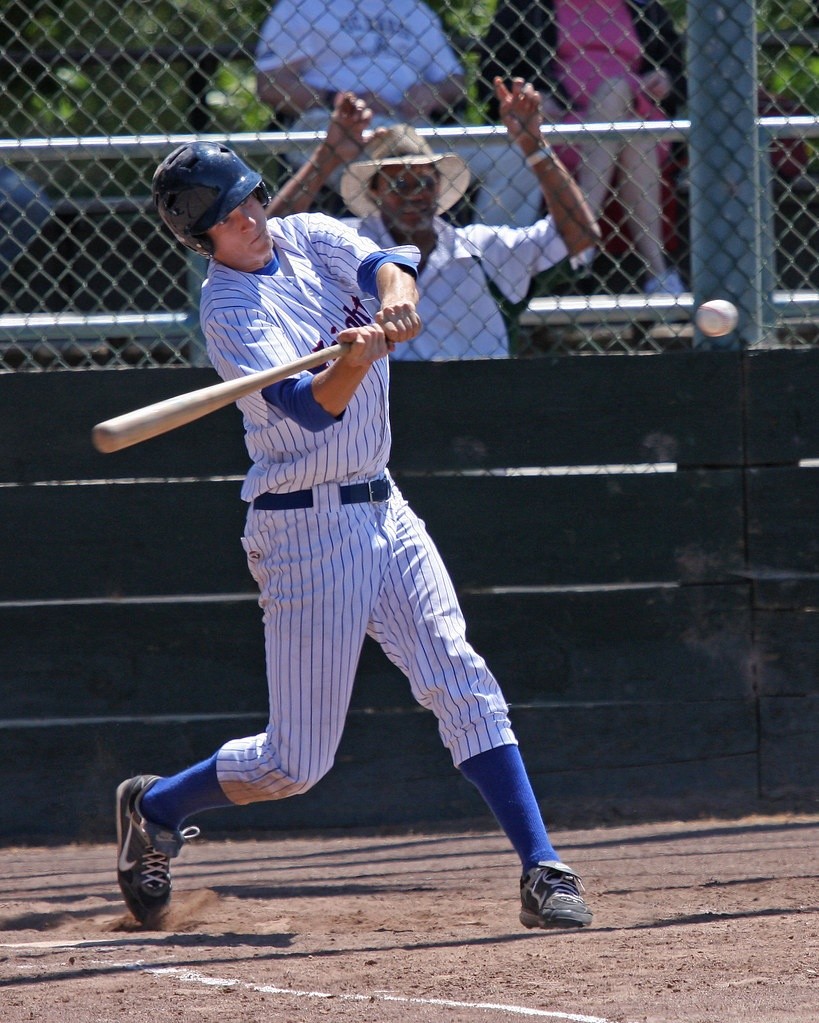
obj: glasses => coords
[384,176,437,193]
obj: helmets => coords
[150,141,271,260]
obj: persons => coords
[254,0,543,227]
[260,74,602,364]
[477,0,689,293]
[115,140,593,928]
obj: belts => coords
[254,474,392,510]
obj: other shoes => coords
[645,275,687,295]
[564,247,594,281]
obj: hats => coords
[339,124,471,218]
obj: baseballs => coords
[696,299,741,338]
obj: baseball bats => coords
[92,342,348,455]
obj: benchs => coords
[0,29,819,369]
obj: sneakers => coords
[114,775,200,924]
[519,860,592,929]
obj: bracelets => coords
[525,147,554,168]
